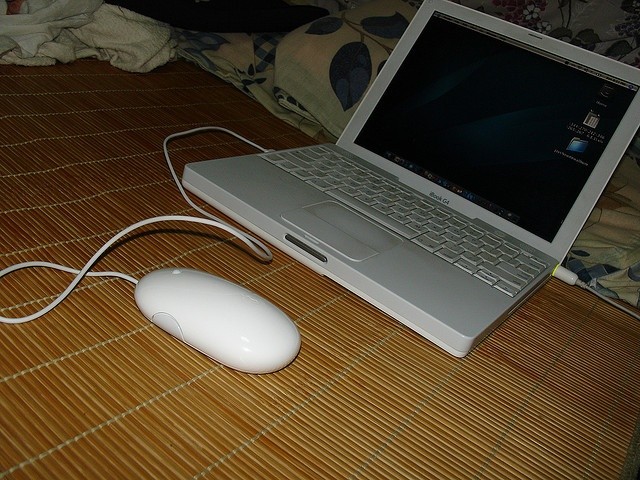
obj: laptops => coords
[180,2,640,358]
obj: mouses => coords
[136,267,302,374]
